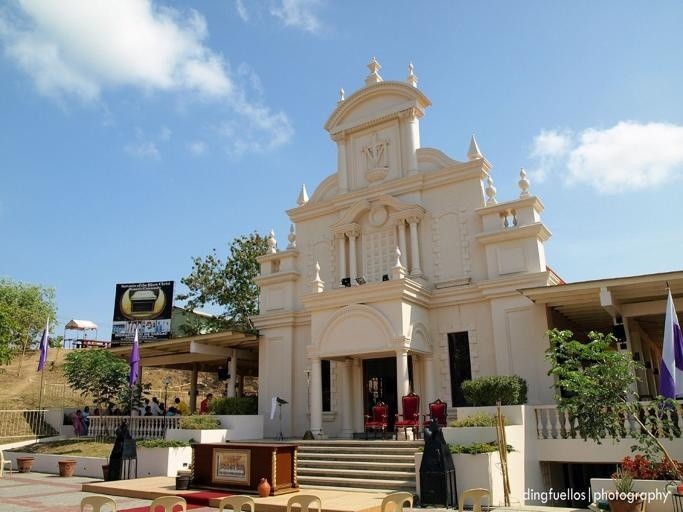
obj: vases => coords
[102,464,109,480]
[17,456,35,472]
[58,460,77,476]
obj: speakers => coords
[217,364,228,381]
[612,324,626,343]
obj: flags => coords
[37,320,49,374]
[129,326,142,389]
[659,291,683,401]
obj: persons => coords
[222,382,228,398]
[235,382,246,398]
[201,392,214,412]
[71,396,184,439]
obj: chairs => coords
[364,400,389,439]
[80,495,116,512]
[287,495,321,512]
[0,447,12,478]
[381,492,414,512]
[395,393,419,440]
[150,496,187,512]
[458,488,491,512]
[219,495,255,512]
[422,399,449,429]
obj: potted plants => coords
[607,464,646,512]
